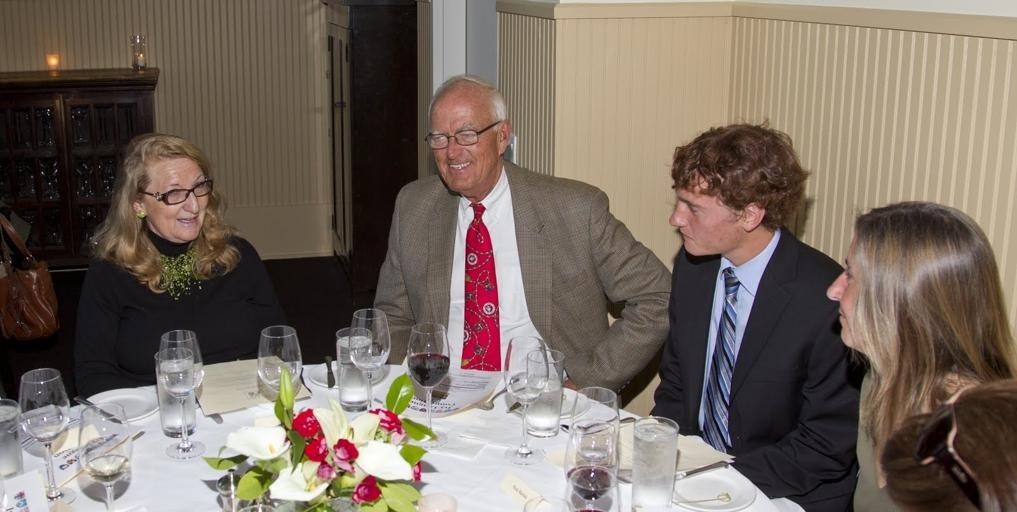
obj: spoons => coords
[559,414,635,435]
[477,375,520,411]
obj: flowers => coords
[203,366,440,512]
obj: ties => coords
[703,268,750,454]
[461,203,501,371]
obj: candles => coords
[48,56,58,71]
[137,53,146,68]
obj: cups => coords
[525,349,566,439]
[153,347,198,437]
[418,491,460,511]
[524,496,570,510]
[335,327,373,411]
[631,416,679,511]
[0,397,25,482]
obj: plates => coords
[308,361,383,389]
[671,472,755,511]
[86,388,159,424]
[558,388,589,420]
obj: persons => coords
[826,202,1017,512]
[649,120,870,512]
[881,377,1017,512]
[373,73,672,400]
[75,132,289,403]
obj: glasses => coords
[139,179,213,205]
[915,383,999,512]
[424,120,500,150]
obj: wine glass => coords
[502,335,546,466]
[18,368,77,508]
[349,308,392,425]
[256,325,302,424]
[78,401,130,511]
[159,329,206,460]
[0,108,117,246]
[563,384,623,512]
[406,322,450,451]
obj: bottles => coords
[217,468,243,512]
[128,33,147,75]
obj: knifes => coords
[324,353,335,389]
[74,395,123,426]
[675,458,730,479]
[503,400,520,414]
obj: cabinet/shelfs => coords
[1,68,160,269]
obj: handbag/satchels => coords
[0,212,61,340]
